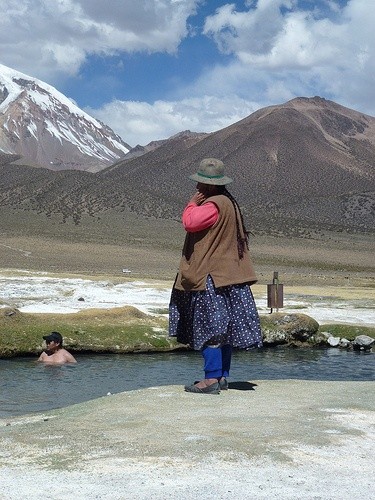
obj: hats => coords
[189,157,233,185]
[43,331,63,343]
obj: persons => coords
[169,158,263,394]
[38,332,77,363]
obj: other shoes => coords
[194,378,228,390]
[184,381,220,394]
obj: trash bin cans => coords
[267,271,283,313]
